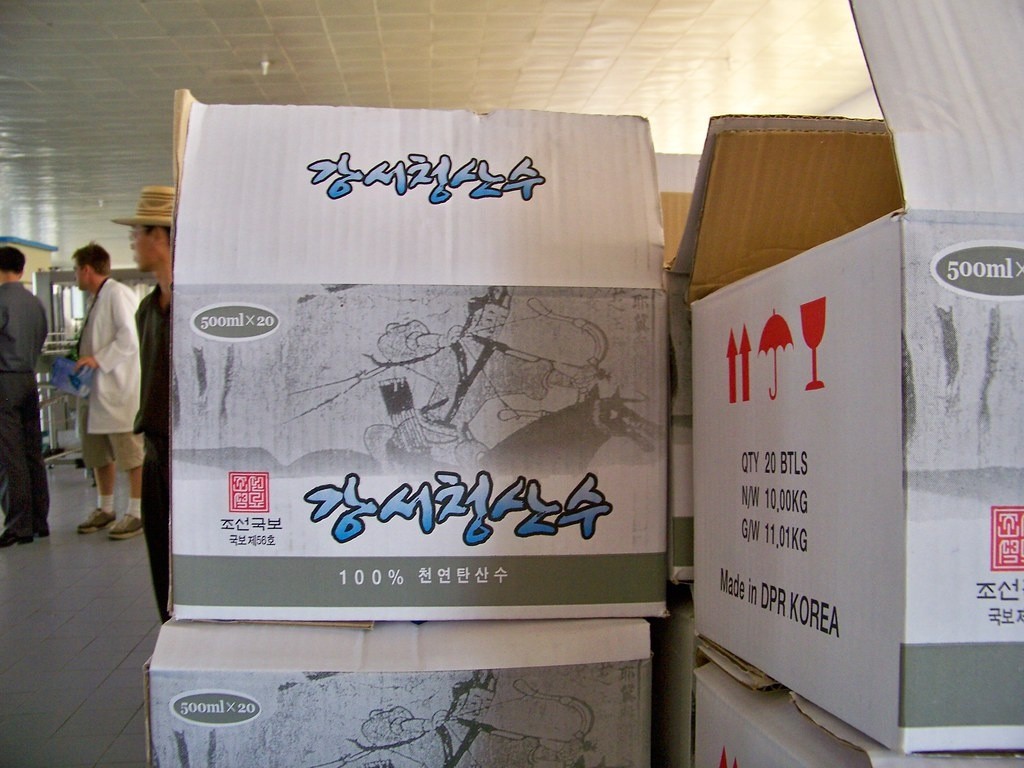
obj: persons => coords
[69,244,147,540]
[110,184,176,622]
[0,247,51,549]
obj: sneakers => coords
[108,514,144,539]
[77,508,115,534]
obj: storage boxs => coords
[142,618,654,768]
[656,115,890,587]
[680,0,1024,759]
[691,645,1024,768]
[165,89,673,629]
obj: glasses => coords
[128,228,157,241]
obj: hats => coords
[110,185,179,226]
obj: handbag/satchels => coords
[48,357,94,399]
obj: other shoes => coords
[33,518,49,537]
[0,529,34,548]
